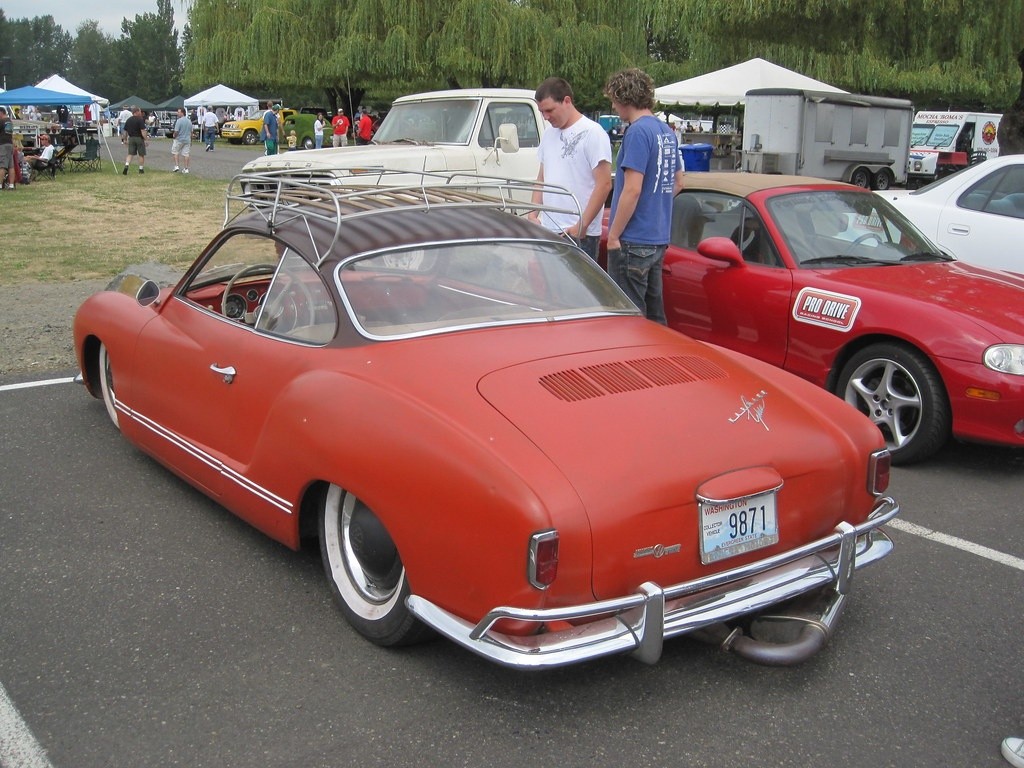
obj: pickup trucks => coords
[221,110,297,146]
[299,106,332,122]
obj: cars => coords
[596,172,1024,468]
[72,161,897,670]
[157,113,198,138]
[278,114,348,150]
[831,153,1024,276]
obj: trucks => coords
[238,87,617,225]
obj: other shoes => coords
[139,169,144,173]
[0,188,2,190]
[206,145,210,151]
[9,188,14,191]
[123,165,128,175]
[211,149,214,151]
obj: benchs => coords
[967,193,1024,219]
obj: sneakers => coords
[173,166,179,172]
[182,168,189,173]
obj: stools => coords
[712,144,731,171]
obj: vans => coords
[908,110,1004,182]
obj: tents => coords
[35,74,259,143]
[0,85,119,174]
[649,57,849,133]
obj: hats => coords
[272,104,283,110]
[38,135,51,140]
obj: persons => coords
[20,134,55,171]
[356,110,372,146]
[526,77,613,264]
[172,107,193,174]
[113,101,297,155]
[601,68,683,327]
[314,112,326,149]
[610,118,705,148]
[332,108,350,147]
[14,108,21,119]
[122,106,148,175]
[0,107,17,189]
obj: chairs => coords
[66,139,102,174]
[670,194,706,249]
[2,162,24,189]
[28,142,77,182]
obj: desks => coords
[681,133,742,172]
[61,127,98,172]
[22,147,45,157]
